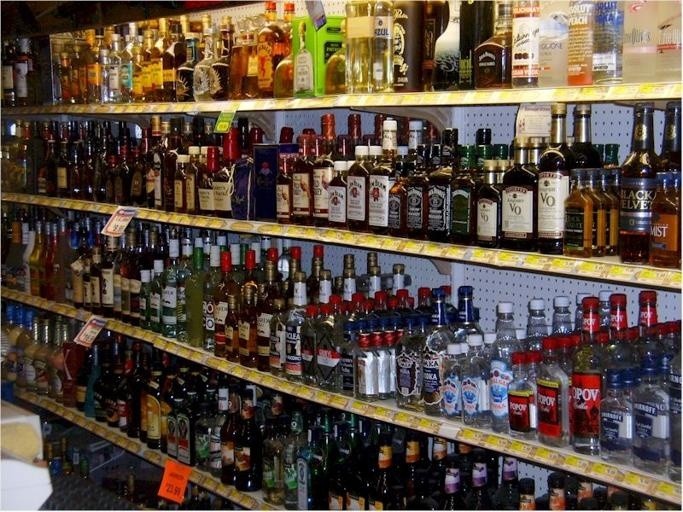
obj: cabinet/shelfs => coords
[1,0,682,511]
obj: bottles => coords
[0,1,681,511]
